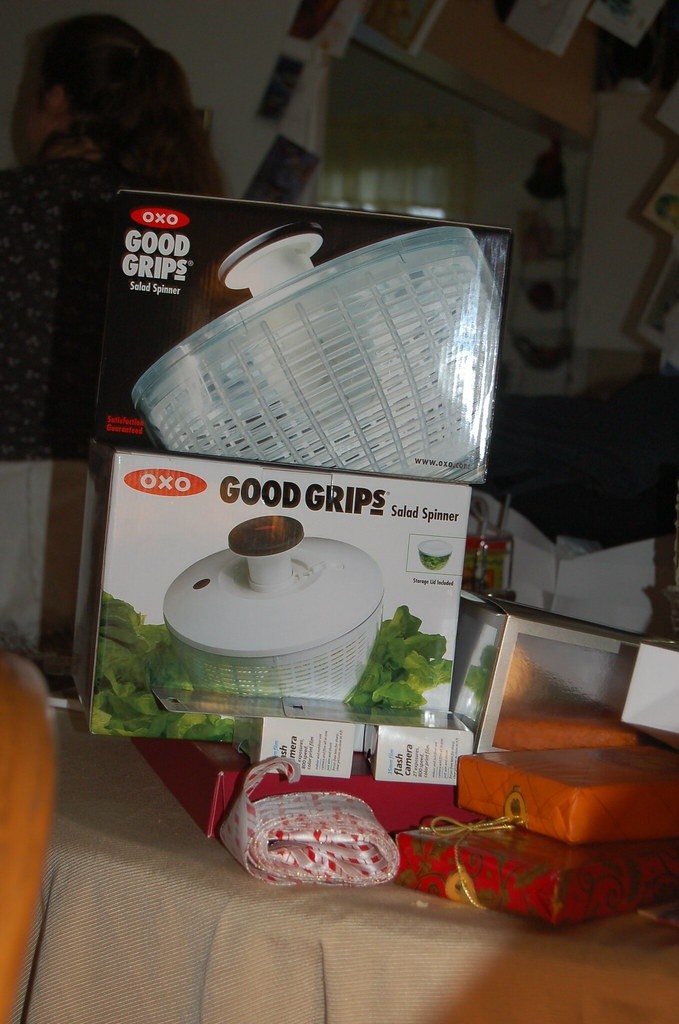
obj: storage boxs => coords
[64,188,679,927]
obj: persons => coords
[0,12,225,462]
